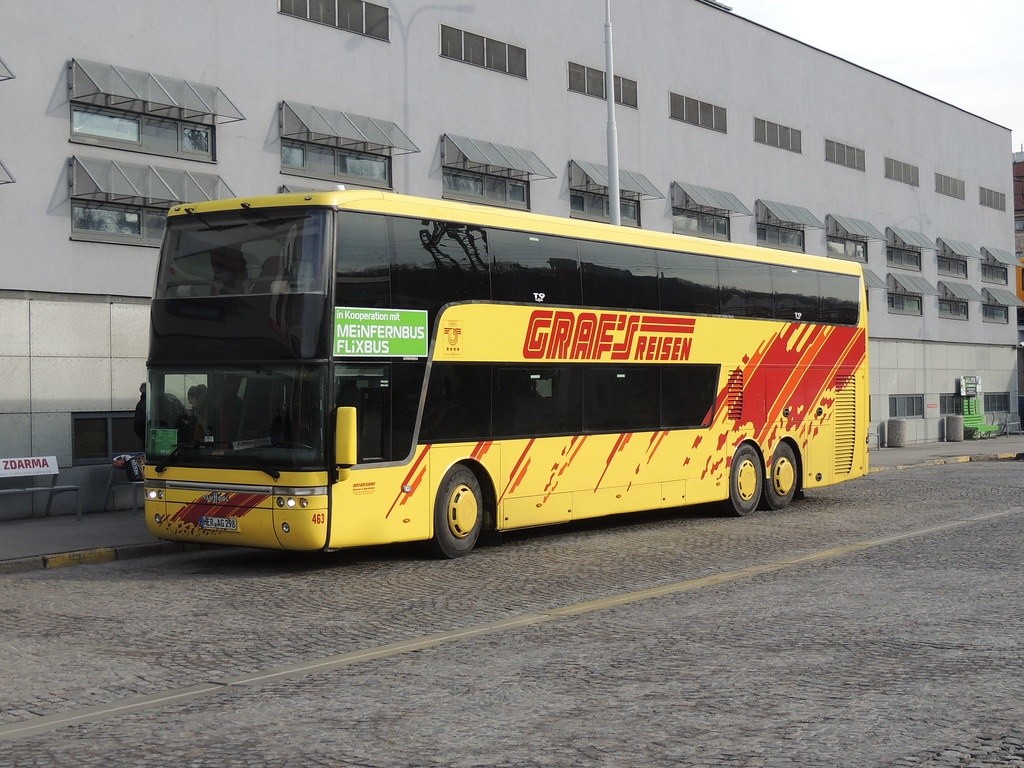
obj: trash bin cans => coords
[887,418,907,448]
[946,415,963,442]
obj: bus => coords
[142,187,870,563]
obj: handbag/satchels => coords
[124,453,145,482]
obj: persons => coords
[134,381,227,452]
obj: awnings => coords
[569,159,666,201]
[983,287,1024,306]
[280,100,421,156]
[441,133,557,180]
[70,155,239,211]
[939,281,988,302]
[981,246,1024,268]
[936,237,987,260]
[862,268,890,290]
[888,272,942,297]
[671,180,754,217]
[825,213,888,242]
[756,199,827,230]
[885,225,941,251]
[71,57,247,125]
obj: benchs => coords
[987,411,1022,437]
[962,415,999,441]
[101,453,144,517]
[0,456,84,522]
